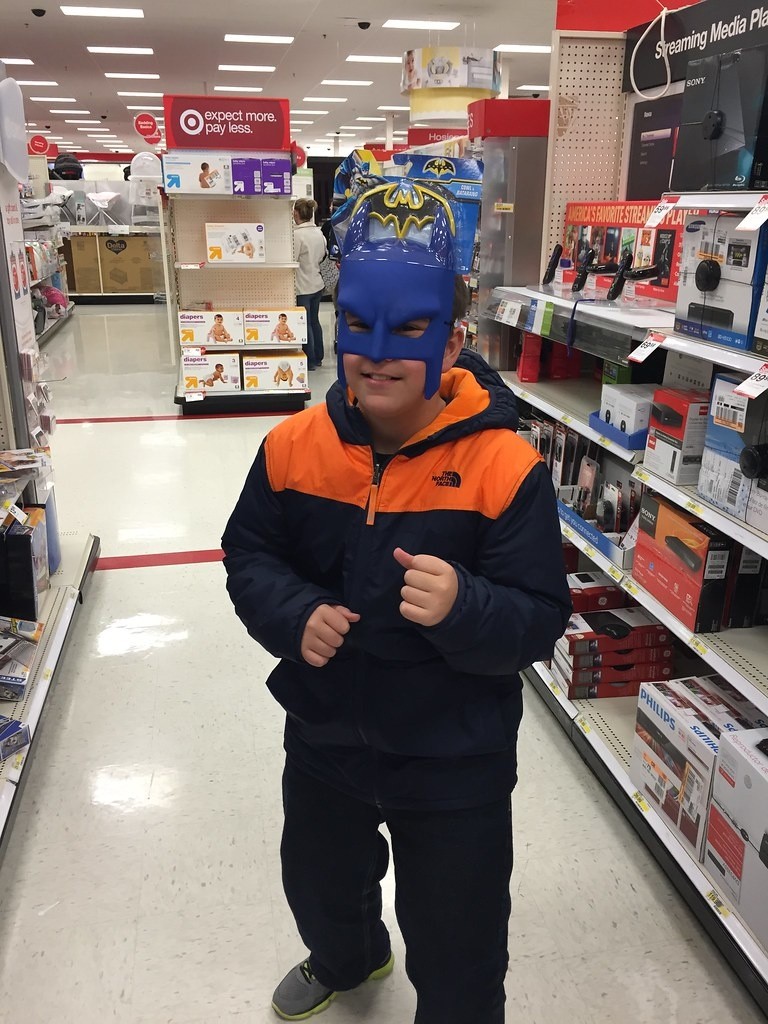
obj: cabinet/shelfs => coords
[167,193,313,415]
[22,181,79,353]
[487,288,768,1015]
[1,159,101,837]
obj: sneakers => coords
[270,946,394,1020]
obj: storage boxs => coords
[0,503,50,763]
[515,191,767,959]
[163,154,309,394]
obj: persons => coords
[199,363,228,387]
[221,181,569,1023]
[207,314,233,343]
[271,314,296,342]
[274,361,294,387]
[403,50,421,90]
[237,242,254,259]
[199,163,217,188]
[292,198,328,371]
